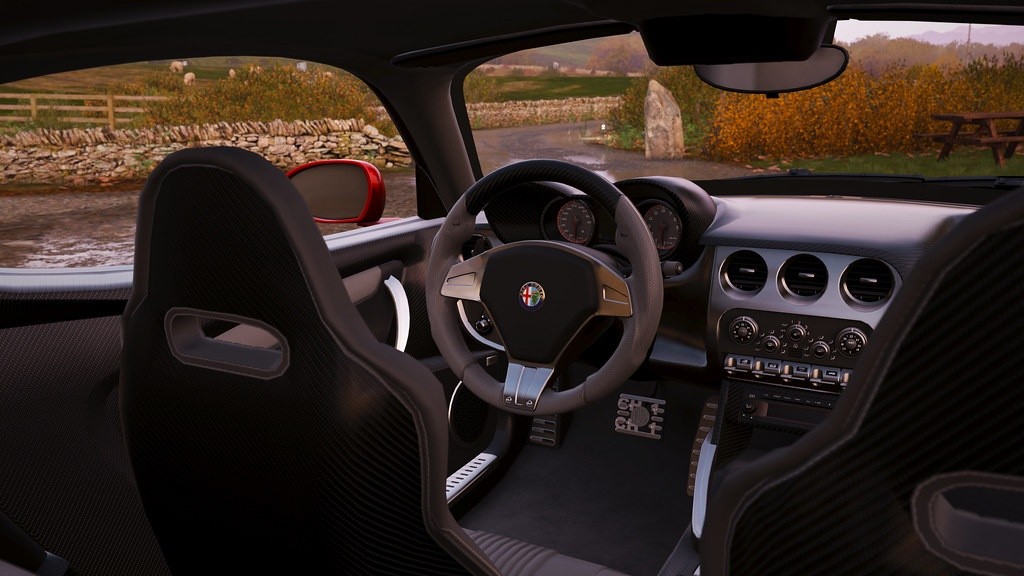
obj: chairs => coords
[697,196,1024,576]
[118,146,625,576]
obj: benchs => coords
[965,136,1024,143]
[916,129,1016,138]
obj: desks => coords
[930,112,1024,166]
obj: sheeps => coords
[229,69,236,78]
[169,62,183,74]
[184,72,196,86]
